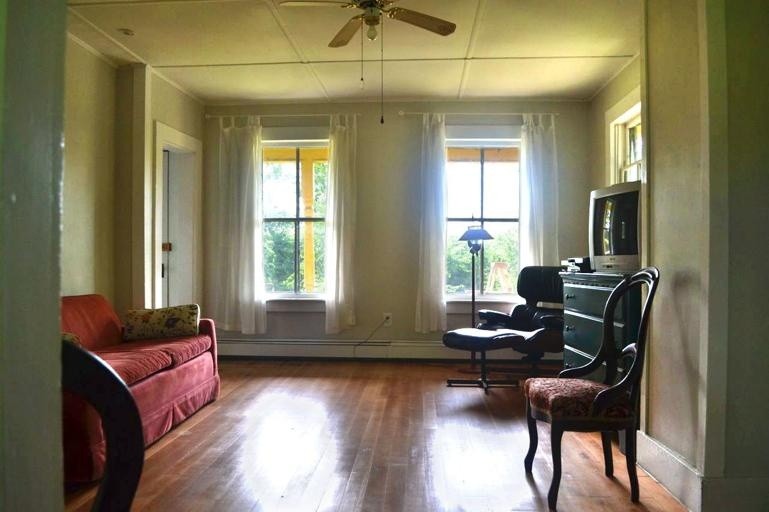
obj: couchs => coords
[59,294,222,493]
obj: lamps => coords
[356,2,389,125]
[456,222,493,373]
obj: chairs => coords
[520,265,659,512]
[440,263,566,394]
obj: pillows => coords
[120,303,201,341]
[60,331,80,343]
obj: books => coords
[537,300,564,310]
[560,255,590,265]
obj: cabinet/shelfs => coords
[558,266,643,456]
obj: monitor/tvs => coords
[589,180,641,274]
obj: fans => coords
[278,1,458,50]
